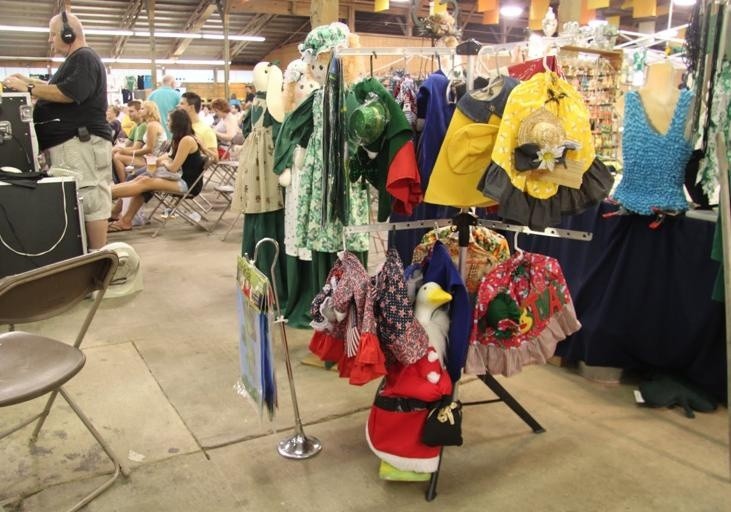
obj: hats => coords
[349,101,385,146]
[298,23,350,60]
[517,106,568,175]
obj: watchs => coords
[25,83,35,96]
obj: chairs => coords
[0,250,130,512]
[148,141,243,241]
[375,0,658,37]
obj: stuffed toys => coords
[409,281,453,370]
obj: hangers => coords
[344,219,530,285]
[356,48,573,93]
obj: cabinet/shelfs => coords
[559,41,619,167]
[345,214,596,502]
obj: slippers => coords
[108,221,133,233]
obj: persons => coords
[227,60,286,214]
[0,7,116,253]
[270,54,322,263]
[103,73,257,235]
[607,59,699,218]
[268,22,367,256]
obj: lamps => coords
[0,25,265,42]
[52,57,231,65]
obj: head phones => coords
[61,11,76,44]
